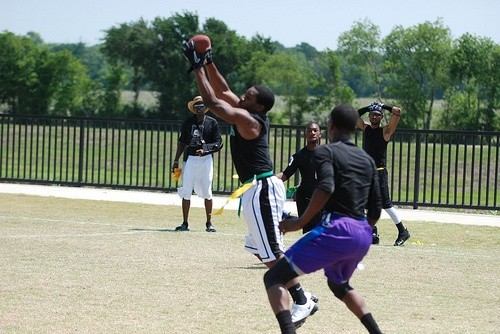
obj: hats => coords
[369,105,383,114]
[188,96,209,114]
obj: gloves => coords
[180,40,211,74]
[367,102,384,112]
[205,49,212,65]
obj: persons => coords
[263,103,382,334]
[180,39,319,332]
[170,95,223,232]
[355,102,410,247]
[275,120,330,234]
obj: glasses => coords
[193,104,204,108]
[370,113,381,116]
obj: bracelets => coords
[173,160,179,164]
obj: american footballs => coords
[187,34,210,57]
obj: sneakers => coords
[206,222,216,232]
[372,226,379,244]
[282,210,291,221]
[394,228,410,246]
[175,222,188,231]
[290,291,318,329]
